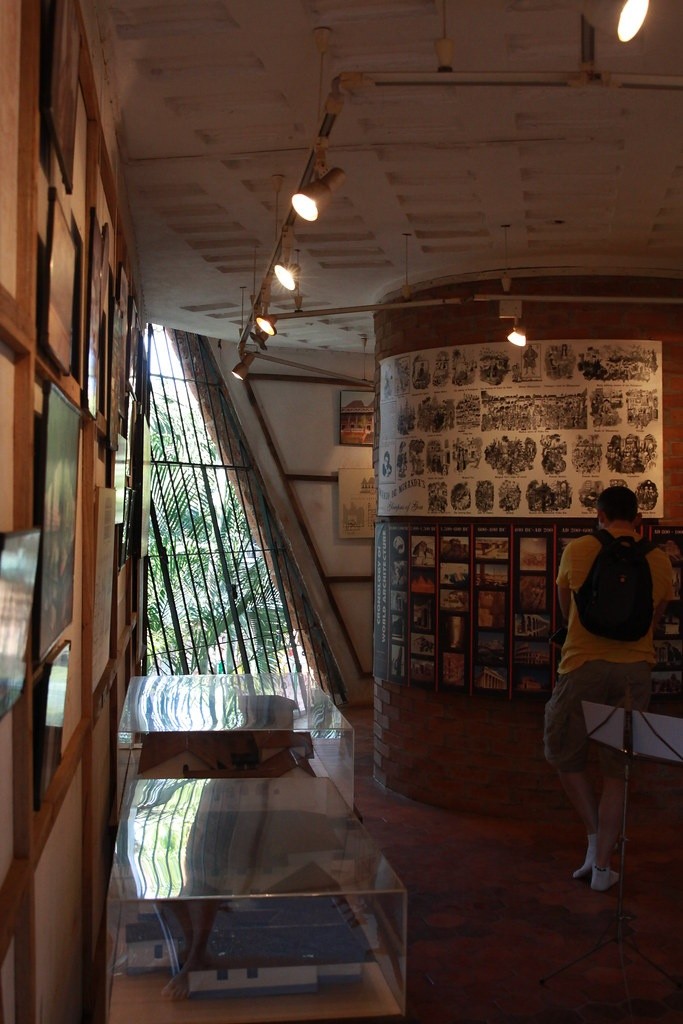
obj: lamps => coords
[506,320,530,348]
[256,313,278,337]
[289,163,349,224]
[273,261,302,292]
[582,0,651,45]
[231,354,256,381]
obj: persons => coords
[543,484,673,892]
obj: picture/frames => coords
[33,382,84,669]
[38,0,140,449]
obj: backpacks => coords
[573,528,657,641]
